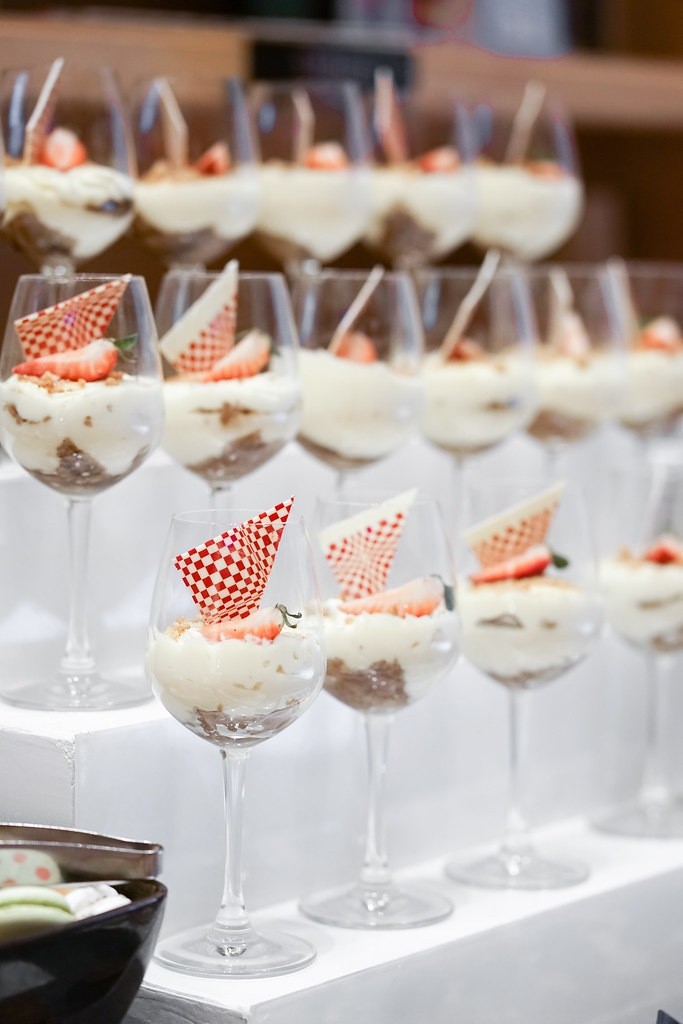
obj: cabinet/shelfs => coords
[0,440,683,1024]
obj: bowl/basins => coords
[0,879,170,1024]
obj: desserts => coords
[0,127,682,753]
[0,881,131,948]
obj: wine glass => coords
[2,263,683,721]
[0,52,588,342]
[151,484,683,977]
